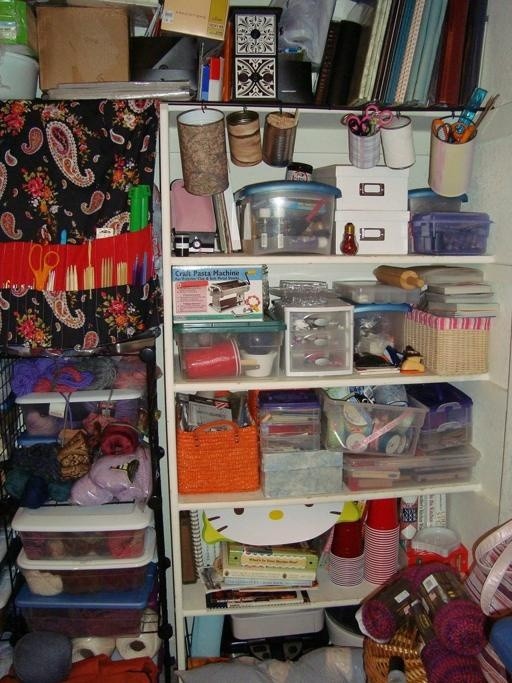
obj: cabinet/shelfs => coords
[0,330,173,682]
[171,255,494,617]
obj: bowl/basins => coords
[322,610,367,650]
[2,47,42,102]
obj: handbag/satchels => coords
[460,520,512,681]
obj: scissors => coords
[28,244,60,291]
[432,117,474,144]
[342,105,393,136]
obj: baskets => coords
[362,621,428,682]
[399,309,489,375]
[177,414,259,495]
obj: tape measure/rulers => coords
[349,407,414,448]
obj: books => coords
[179,512,222,583]
[158,0,490,109]
[415,267,500,320]
[221,546,316,588]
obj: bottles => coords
[175,234,190,256]
[339,222,357,254]
[285,161,314,183]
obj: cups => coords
[347,115,379,170]
[380,114,415,170]
[425,116,477,199]
[261,110,298,166]
[329,497,401,587]
[176,107,230,195]
[181,332,279,378]
[226,110,263,167]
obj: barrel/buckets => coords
[325,609,364,647]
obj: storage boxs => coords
[11,501,158,638]
[16,351,146,438]
[232,164,494,254]
[255,382,481,499]
[36,6,131,92]
[173,265,411,378]
